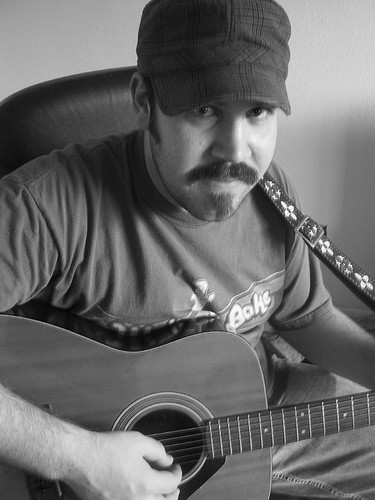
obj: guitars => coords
[0,312,375,496]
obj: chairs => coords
[0,66,145,177]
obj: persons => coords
[0,1,375,500]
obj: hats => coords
[136,1,292,116]
